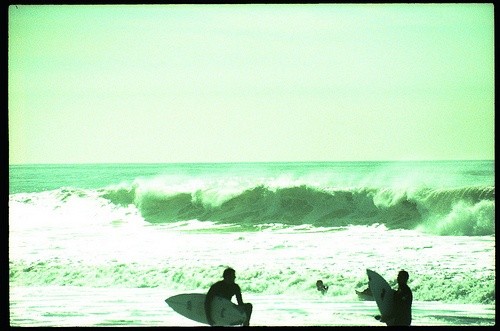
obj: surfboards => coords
[165,294,246,327]
[355,290,374,301]
[367,268,392,317]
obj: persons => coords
[205,268,253,327]
[375,271,413,326]
[316,280,329,295]
